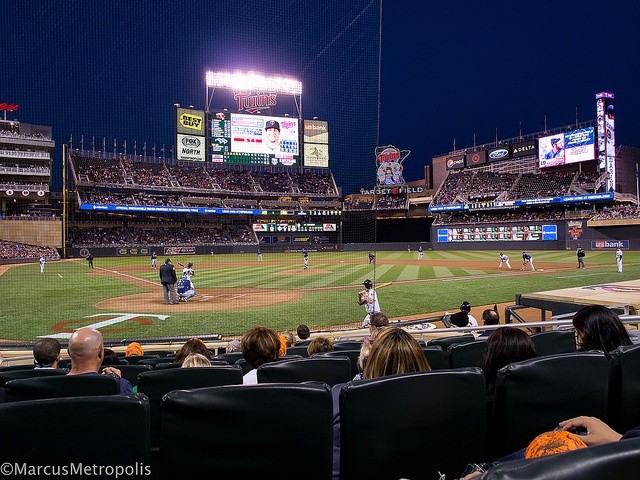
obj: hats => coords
[551,137,564,146]
[265,119,281,133]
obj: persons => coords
[151,252,157,266]
[572,305,634,353]
[177,262,195,303]
[87,253,93,267]
[369,252,376,264]
[159,257,177,304]
[40,256,45,272]
[481,308,501,337]
[303,250,308,269]
[521,253,535,271]
[298,324,311,341]
[616,247,623,273]
[417,245,423,260]
[576,244,587,269]
[306,337,335,356]
[330,327,431,480]
[257,249,262,260]
[498,253,513,269]
[34,338,61,369]
[282,331,295,349]
[358,279,381,328]
[177,338,213,366]
[450,301,479,330]
[545,139,565,159]
[67,328,132,392]
[241,326,282,385]
[452,416,639,480]
[363,314,390,337]
[263,121,282,154]
[482,328,540,397]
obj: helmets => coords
[460,300,472,312]
[363,279,374,289]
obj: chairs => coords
[333,342,362,351]
[137,365,243,403]
[138,358,175,364]
[427,334,475,348]
[421,346,445,370]
[159,381,334,480]
[488,352,609,460]
[449,340,488,369]
[0,392,152,479]
[530,330,576,357]
[235,356,294,375]
[485,436,640,479]
[310,350,360,378]
[609,342,640,435]
[286,347,309,360]
[339,367,489,479]
[118,355,160,364]
[3,374,120,405]
[210,354,244,365]
[257,355,352,386]
[0,369,70,386]
[102,364,153,382]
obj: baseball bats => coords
[358,282,392,294]
[177,263,186,268]
